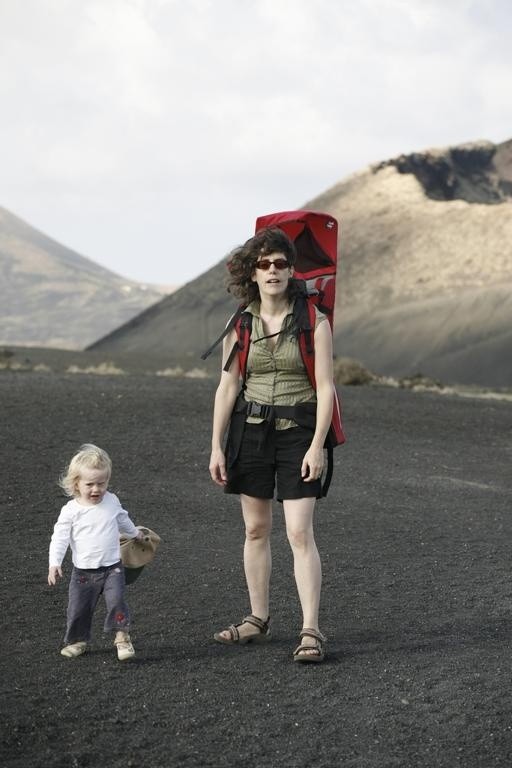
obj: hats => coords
[120,526,160,584]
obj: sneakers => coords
[60,641,88,657]
[115,633,134,660]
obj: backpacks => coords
[237,209,348,451]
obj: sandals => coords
[214,614,275,644]
[295,627,325,665]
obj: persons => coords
[45,442,147,662]
[205,223,338,665]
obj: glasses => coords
[255,260,292,271]
